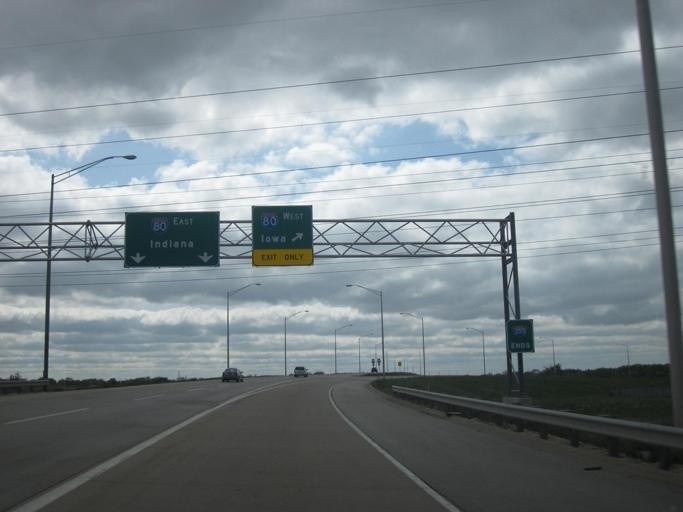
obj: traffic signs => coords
[126,212,220,267]
[252,205,315,267]
[506,319,535,353]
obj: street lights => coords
[335,321,354,374]
[351,330,429,373]
[616,342,631,366]
[44,153,138,380]
[466,327,486,375]
[344,281,387,380]
[284,309,309,376]
[226,281,263,369]
[541,336,556,367]
[398,309,428,376]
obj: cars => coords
[221,367,244,383]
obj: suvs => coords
[293,365,309,378]
